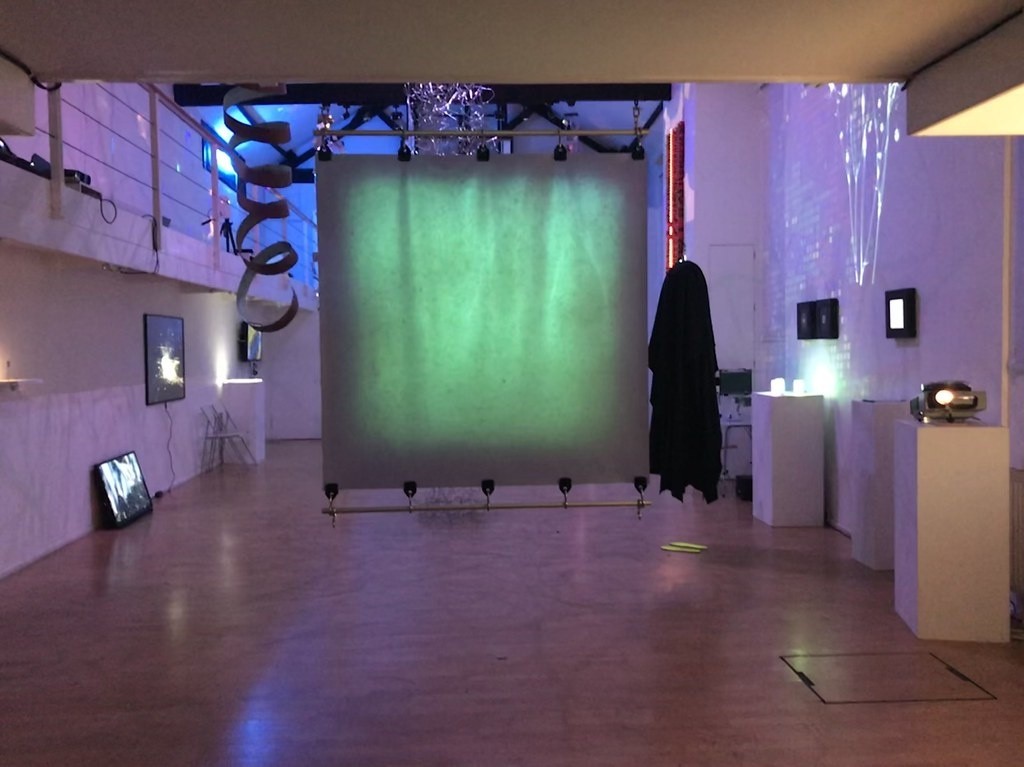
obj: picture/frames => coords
[885,289,916,339]
[662,120,685,270]
[795,298,839,342]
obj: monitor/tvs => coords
[240,322,261,362]
[95,451,153,529]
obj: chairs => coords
[198,401,257,481]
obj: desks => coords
[720,420,755,489]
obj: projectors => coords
[909,389,986,423]
